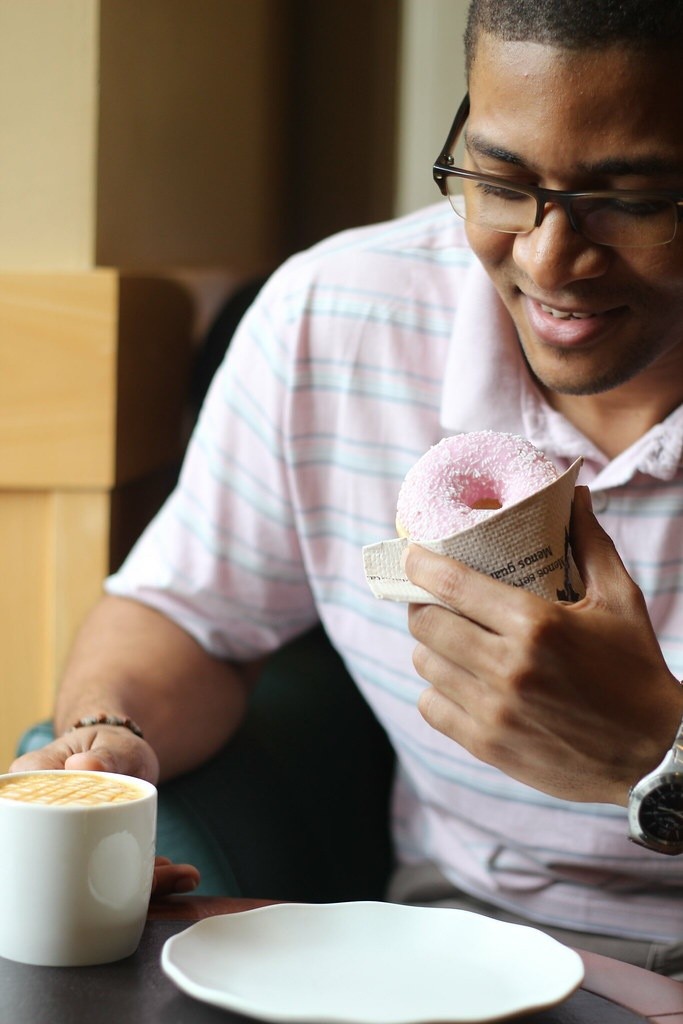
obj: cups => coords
[1,771,159,970]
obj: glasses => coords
[432,91,683,248]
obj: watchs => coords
[628,711,683,856]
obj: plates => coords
[160,901,586,1024]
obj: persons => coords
[8,0,683,982]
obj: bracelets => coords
[62,713,144,740]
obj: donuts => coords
[394,430,559,543]
